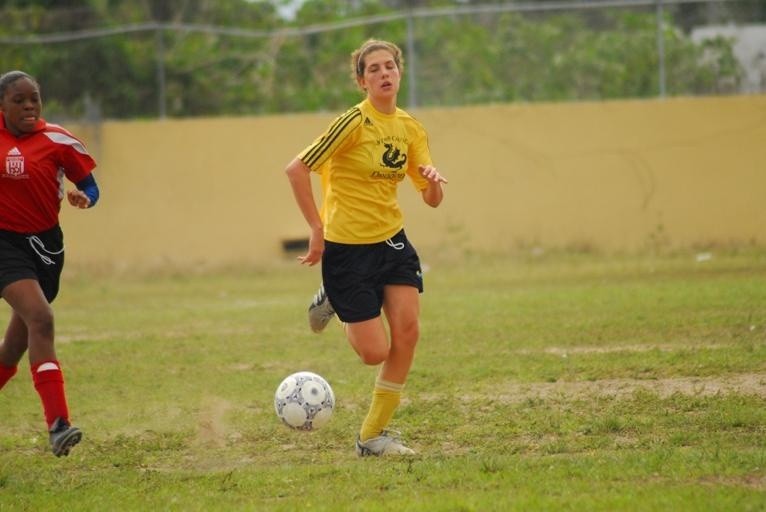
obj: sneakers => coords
[355,428,416,456]
[49,417,83,458]
[308,282,335,335]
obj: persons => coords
[286,38,449,458]
[0,70,103,459]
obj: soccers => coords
[274,371,334,432]
